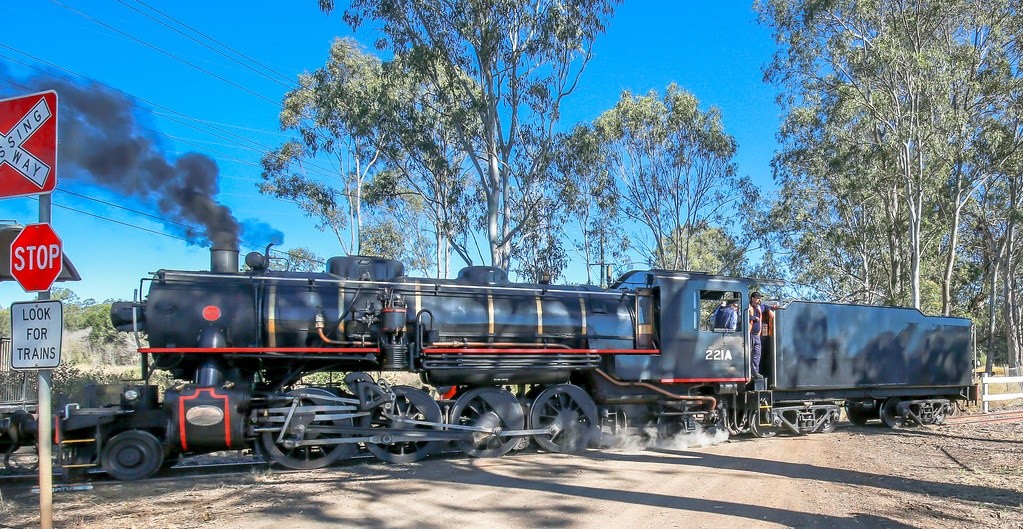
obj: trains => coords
[0,238,987,483]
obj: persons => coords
[710,298,739,331]
[749,292,782,379]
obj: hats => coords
[727,299,739,304]
[751,292,764,297]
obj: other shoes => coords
[753,373,764,379]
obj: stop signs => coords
[9,222,65,293]
